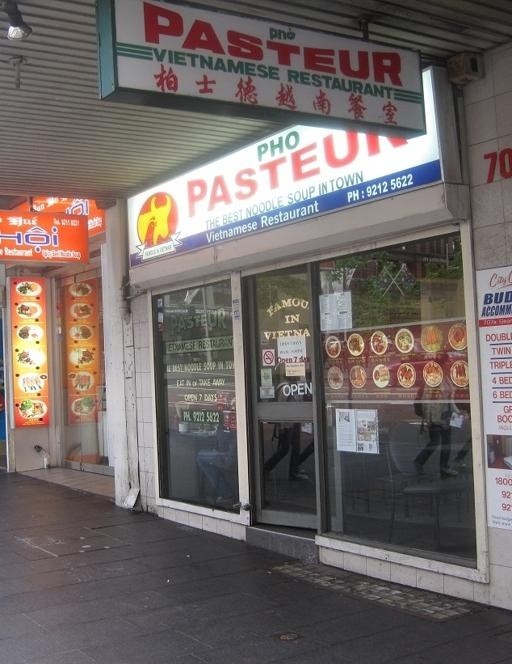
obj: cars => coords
[382,352,466,388]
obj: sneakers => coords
[413,458,427,475]
[215,495,235,503]
[453,454,466,467]
[288,471,309,480]
[440,469,458,476]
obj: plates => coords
[325,323,470,390]
[16,281,48,420]
[69,282,98,417]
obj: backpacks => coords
[414,381,428,435]
[267,384,285,424]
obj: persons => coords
[196,433,238,504]
[413,352,457,477]
[455,438,473,467]
[265,364,308,481]
